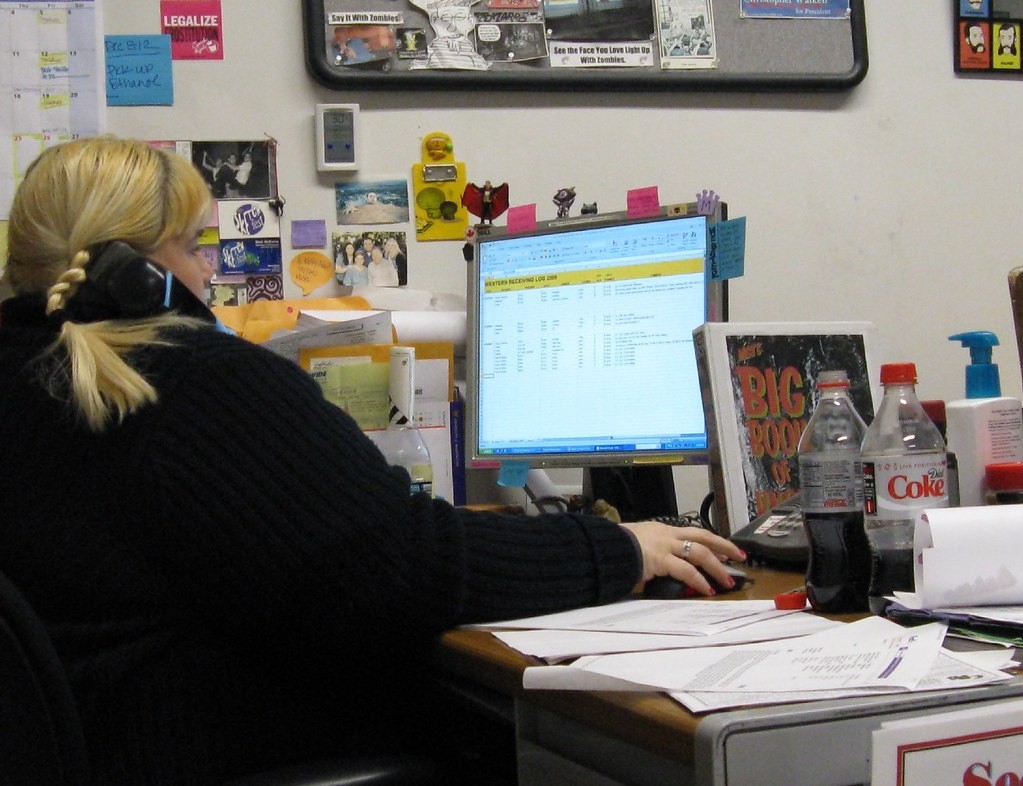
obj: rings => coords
[682,539,691,558]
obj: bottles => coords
[798,371,869,616]
[372,420,433,498]
[984,462,1023,504]
[899,400,960,507]
[859,363,949,617]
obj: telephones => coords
[74,237,219,325]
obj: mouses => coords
[642,560,746,601]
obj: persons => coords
[471,181,504,224]
[331,27,375,65]
[202,147,253,197]
[336,232,407,286]
[0,137,746,786]
[367,191,377,202]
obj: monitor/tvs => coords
[463,201,729,527]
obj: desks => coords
[410,560,1022,786]
